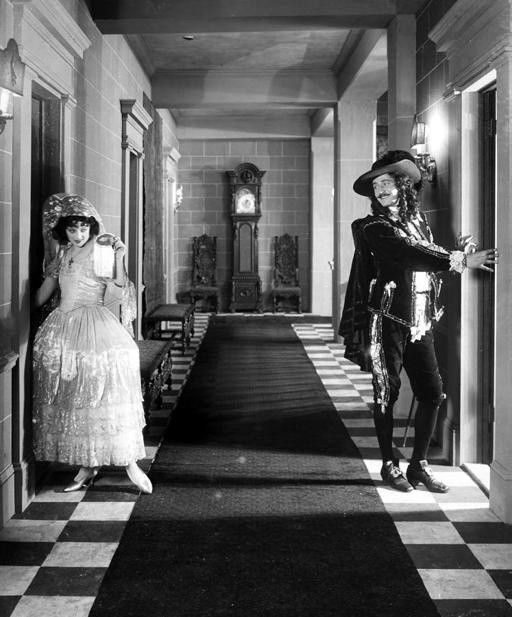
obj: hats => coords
[353,158,421,197]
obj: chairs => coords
[188,232,220,314]
[267,233,307,315]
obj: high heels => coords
[406,460,449,494]
[126,465,152,493]
[381,464,414,492]
[64,467,97,491]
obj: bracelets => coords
[464,255,468,268]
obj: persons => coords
[33,193,153,494]
[337,152,499,493]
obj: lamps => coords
[410,113,437,184]
[0,37,26,133]
[173,183,184,214]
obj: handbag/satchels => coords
[93,232,115,278]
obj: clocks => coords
[224,162,269,315]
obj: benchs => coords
[150,302,196,352]
[133,338,177,417]
